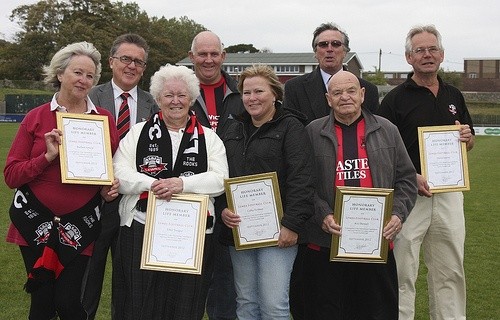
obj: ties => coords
[116,92,131,141]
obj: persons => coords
[213,63,317,320]
[302,69,419,320]
[283,21,381,127]
[3,41,122,320]
[79,30,159,320]
[110,62,231,320]
[376,22,477,320]
[186,29,240,134]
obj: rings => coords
[164,187,168,191]
[396,227,400,233]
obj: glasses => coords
[411,46,441,55]
[112,55,147,67]
[314,39,348,49]
